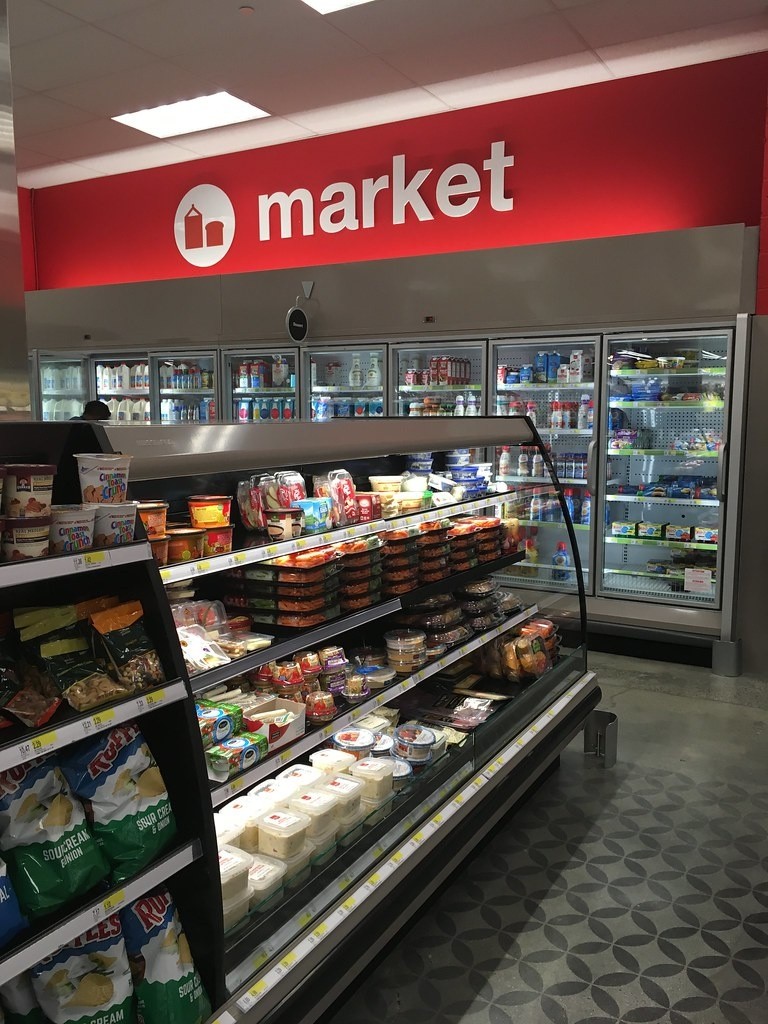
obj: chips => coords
[44,952,118,1009]
[161,928,193,964]
[14,793,73,829]
[113,765,166,798]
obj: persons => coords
[68,401,111,420]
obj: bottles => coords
[365,353,382,386]
[160,363,214,390]
[160,397,216,421]
[42,397,88,421]
[498,442,589,480]
[96,363,150,389]
[506,527,571,580]
[348,353,363,387]
[496,394,594,430]
[503,483,591,526]
[41,366,81,391]
[605,502,611,526]
[607,456,613,480]
[408,395,482,418]
[98,396,150,423]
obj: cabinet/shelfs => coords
[30,312,752,678]
[0,415,620,1024]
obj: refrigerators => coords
[30,313,751,640]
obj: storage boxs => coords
[354,494,381,522]
[646,548,717,581]
[611,520,718,543]
[242,698,306,753]
[289,496,333,535]
